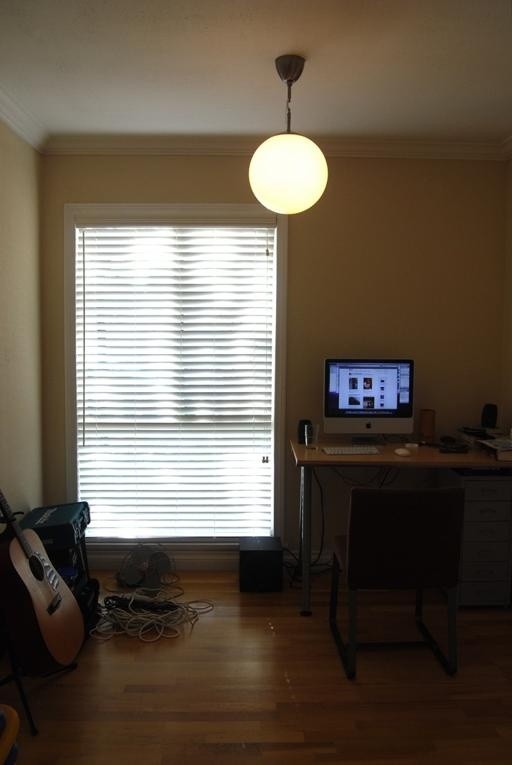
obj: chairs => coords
[325,486,464,680]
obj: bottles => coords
[420,409,436,443]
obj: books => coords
[476,438,512,461]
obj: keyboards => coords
[322,445,381,455]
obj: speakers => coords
[298,420,313,444]
[481,403,498,428]
[239,537,283,592]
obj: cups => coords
[305,424,319,449]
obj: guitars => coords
[2,493,87,673]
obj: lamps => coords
[248,53,330,216]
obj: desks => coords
[289,437,511,617]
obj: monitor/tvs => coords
[322,354,415,444]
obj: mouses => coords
[394,449,411,456]
